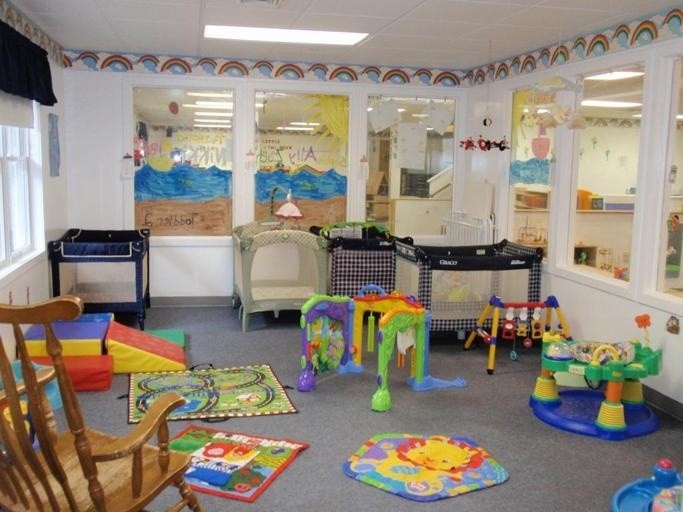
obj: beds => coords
[311,222,413,300]
[392,239,544,340]
[398,210,492,299]
[48,228,150,331]
[232,219,328,333]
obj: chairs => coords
[0,295,205,511]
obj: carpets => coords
[168,426,310,502]
[344,433,510,501]
[127,364,298,424]
